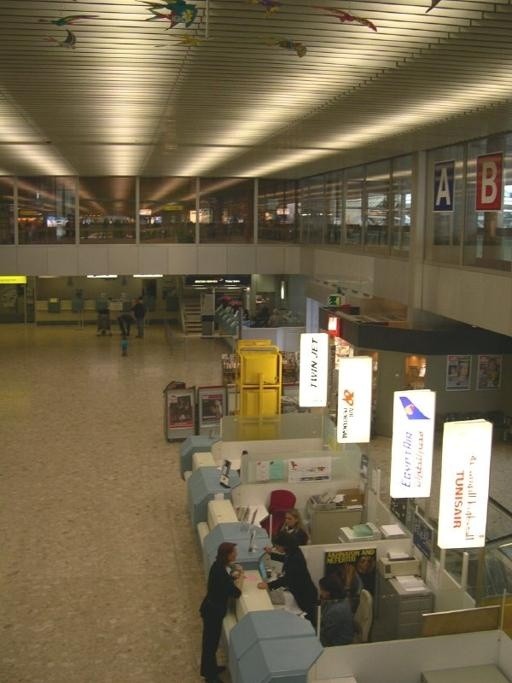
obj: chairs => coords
[317,563,375,643]
[260,490,298,538]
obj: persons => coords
[278,510,308,545]
[199,543,244,683]
[9,214,193,244]
[319,577,354,646]
[242,295,286,326]
[484,359,499,384]
[95,297,147,355]
[330,555,372,598]
[208,399,223,415]
[169,401,192,425]
[449,361,469,386]
[258,532,317,623]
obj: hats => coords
[219,542,236,553]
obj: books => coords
[396,575,425,591]
[379,525,404,538]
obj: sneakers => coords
[200,664,225,683]
